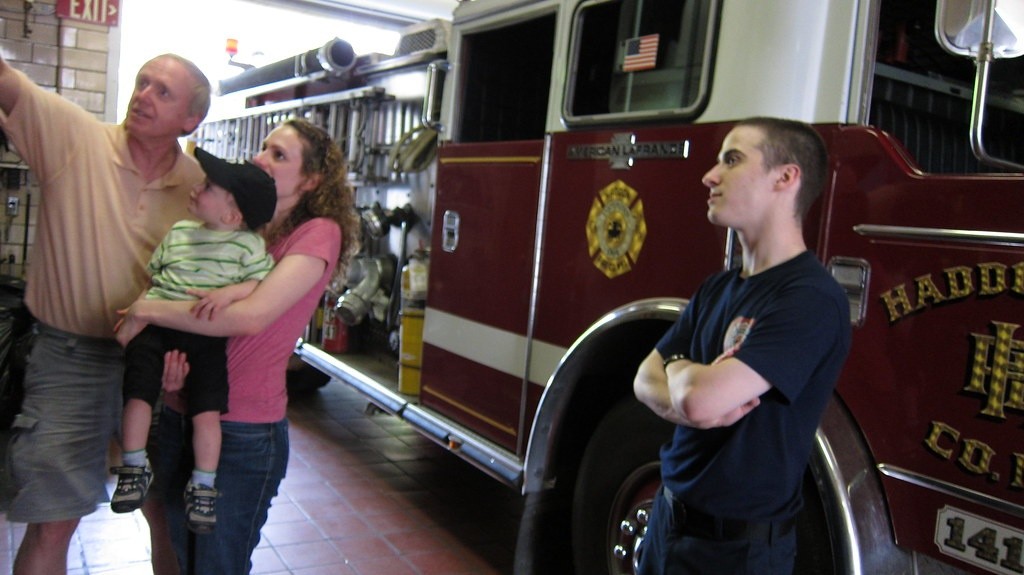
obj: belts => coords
[663,486,797,541]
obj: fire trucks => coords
[182,0,1024,574]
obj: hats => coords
[195,147,277,230]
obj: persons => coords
[634,117,851,575]
[113,120,357,575]
[110,146,277,527]
[0,56,210,575]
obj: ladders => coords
[197,86,386,188]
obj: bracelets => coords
[663,353,687,372]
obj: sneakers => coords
[183,479,218,533]
[110,459,154,514]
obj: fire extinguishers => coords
[323,292,348,354]
